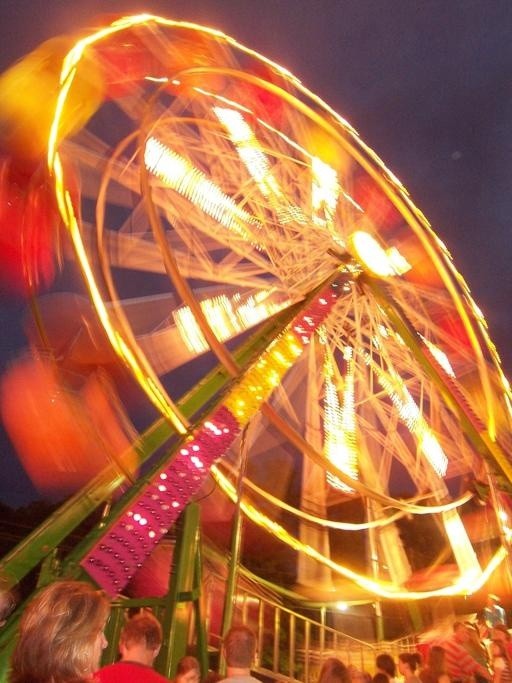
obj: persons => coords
[0,573,263,683]
[317,593,512,683]
